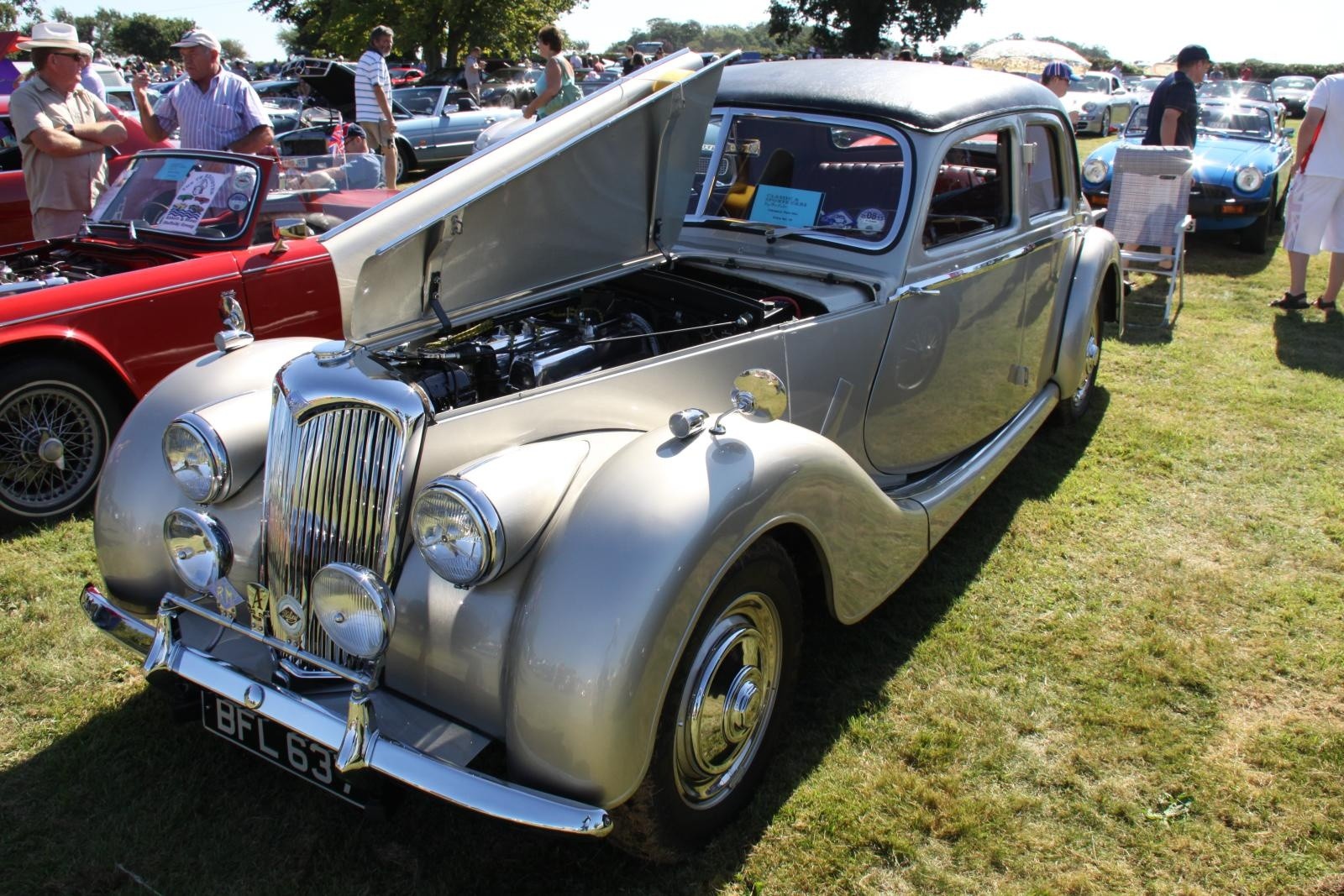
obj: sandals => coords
[1268,293,1310,309]
[1313,296,1336,313]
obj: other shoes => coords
[1154,266,1172,273]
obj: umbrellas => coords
[972,42,1092,77]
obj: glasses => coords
[47,51,81,62]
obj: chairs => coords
[1110,145,1191,333]
[1229,115,1262,129]
[408,96,432,113]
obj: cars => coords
[1196,80,1287,129]
[692,118,998,192]
[1122,75,1165,106]
[1082,98,1294,256]
[1270,75,1317,120]
[0,149,402,532]
[1059,70,1141,138]
[0,57,623,248]
[79,47,1127,860]
[635,42,663,65]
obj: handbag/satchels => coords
[544,58,582,114]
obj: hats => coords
[342,122,367,139]
[15,22,92,56]
[1043,63,1083,82]
[1178,45,1214,64]
[170,29,221,53]
[78,43,94,58]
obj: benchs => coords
[812,157,1007,240]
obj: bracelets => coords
[224,147,232,152]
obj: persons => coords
[220,58,280,80]
[355,25,397,190]
[297,80,316,106]
[465,46,486,107]
[1112,61,1252,80]
[327,123,344,165]
[617,46,665,77]
[7,22,129,239]
[132,29,275,224]
[154,59,183,82]
[1121,44,1210,275]
[419,53,447,73]
[115,62,124,79]
[708,46,971,66]
[339,55,344,62]
[523,26,574,122]
[123,56,157,77]
[524,51,606,80]
[1268,73,1344,309]
[288,50,295,61]
[94,48,113,67]
[298,123,380,189]
[1025,62,1084,216]
[78,42,106,103]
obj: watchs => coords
[64,124,75,136]
